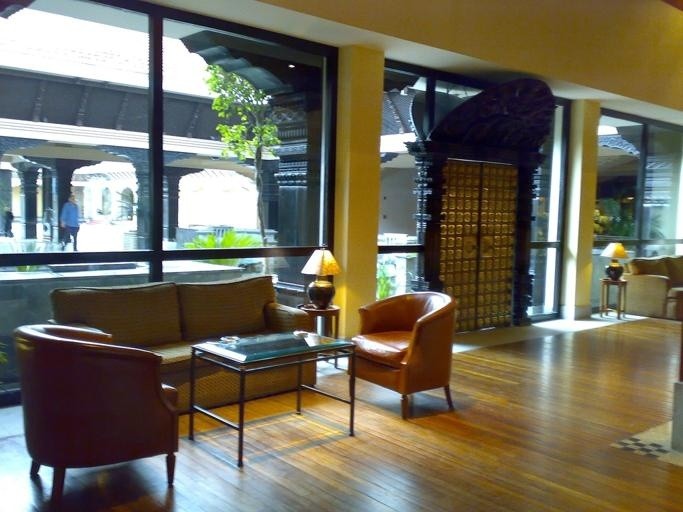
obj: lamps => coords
[599,243,629,280]
[300,248,342,309]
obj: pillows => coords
[664,256,683,284]
[630,259,669,277]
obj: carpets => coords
[608,421,683,468]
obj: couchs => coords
[347,291,456,419]
[619,255,683,319]
[14,325,177,512]
[47,275,317,414]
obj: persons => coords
[60,192,80,251]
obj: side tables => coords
[300,305,339,368]
[601,280,626,319]
